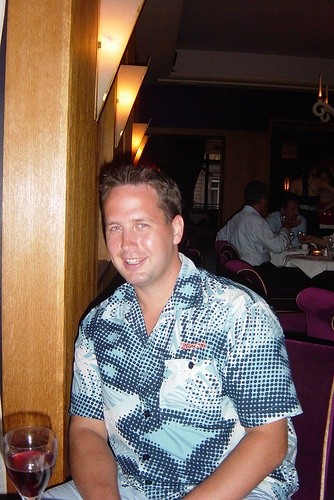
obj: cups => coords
[313,249,321,256]
[302,244,308,249]
[280,215,288,224]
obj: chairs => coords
[216,240,334,344]
[285,339,334,500]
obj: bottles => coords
[323,248,328,256]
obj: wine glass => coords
[3,426,59,500]
[287,229,306,249]
[326,237,334,257]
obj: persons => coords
[216,180,334,288]
[39,166,304,500]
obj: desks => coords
[269,247,310,267]
[285,256,334,279]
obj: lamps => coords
[94,0,145,124]
[134,135,147,160]
[114,66,148,149]
[132,123,147,157]
[312,72,334,123]
